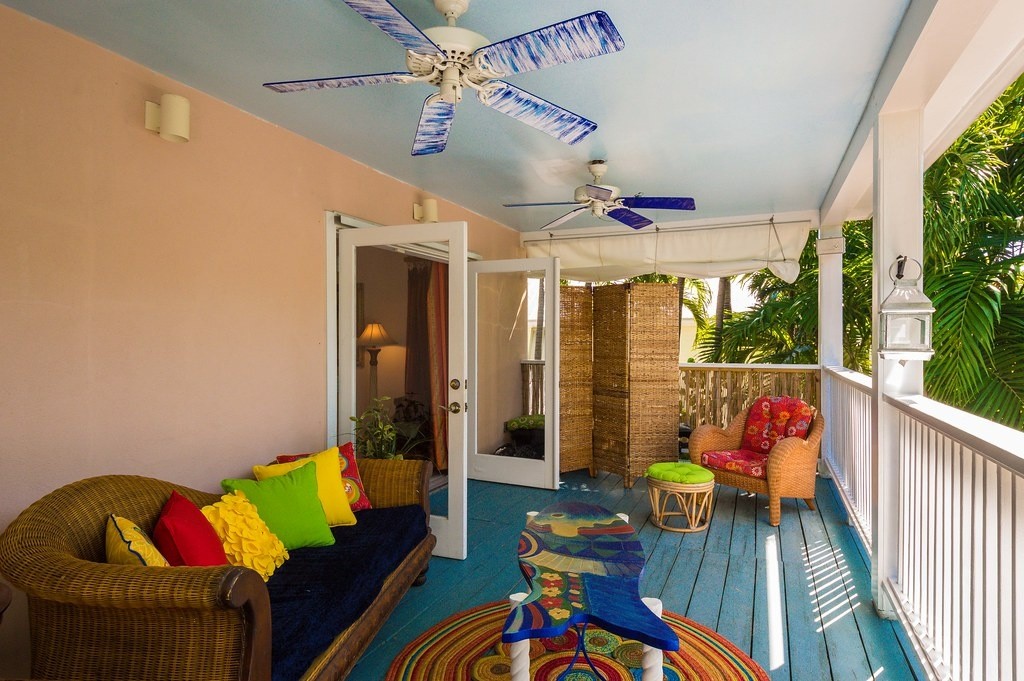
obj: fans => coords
[498,159,695,232]
[259,0,628,156]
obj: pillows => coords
[198,489,291,584]
[105,514,172,568]
[253,445,357,527]
[276,442,373,513]
[221,460,336,551]
[153,490,228,568]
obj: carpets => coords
[377,596,773,681]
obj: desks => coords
[499,501,679,681]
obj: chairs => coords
[688,394,825,526]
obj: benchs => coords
[0,440,438,681]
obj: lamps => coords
[352,318,398,423]
[144,93,191,144]
[874,250,937,361]
[413,198,438,226]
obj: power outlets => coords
[505,421,512,434]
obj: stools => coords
[642,461,717,533]
